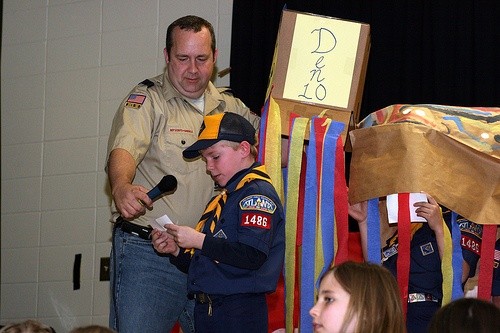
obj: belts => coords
[191,292,266,304]
[403,292,436,303]
[115,217,153,239]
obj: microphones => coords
[116,175,177,222]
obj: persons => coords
[151,112,287,333]
[104,15,290,333]
[0,186,500,333]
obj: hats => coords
[182,112,257,159]
